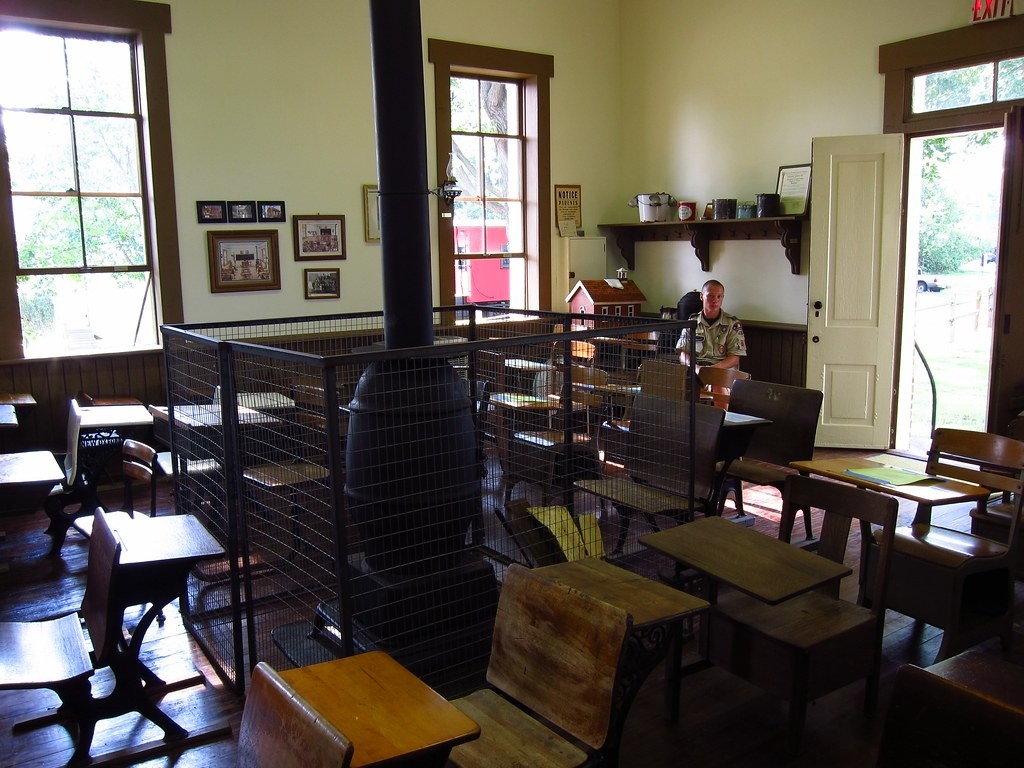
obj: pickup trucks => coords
[917,268,950,292]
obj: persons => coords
[674,280,748,412]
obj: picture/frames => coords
[293,214,346,261]
[363,183,381,243]
[304,268,341,299]
[207,229,281,293]
[197,201,286,224]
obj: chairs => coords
[0,324,1024,768]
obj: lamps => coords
[431,152,464,206]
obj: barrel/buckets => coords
[756,193,780,218]
[712,198,737,220]
[636,192,669,223]
[677,201,697,221]
